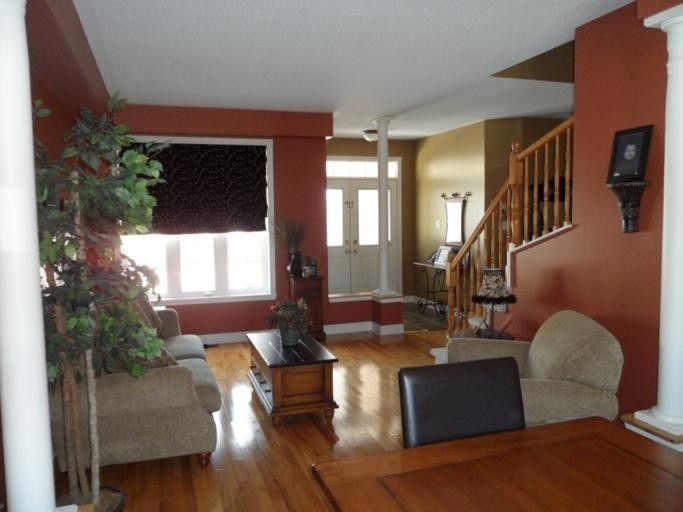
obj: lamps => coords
[363,129,378,143]
[471,267,517,338]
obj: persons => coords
[612,140,639,176]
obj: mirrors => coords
[444,197,466,247]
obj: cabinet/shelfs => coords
[287,275,326,342]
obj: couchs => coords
[44,289,222,473]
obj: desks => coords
[413,262,470,321]
[310,415,683,512]
[445,327,515,340]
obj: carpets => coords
[405,301,449,330]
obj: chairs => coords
[398,356,525,449]
[446,308,624,429]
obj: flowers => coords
[264,298,315,336]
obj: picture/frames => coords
[607,125,653,182]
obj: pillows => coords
[103,339,178,374]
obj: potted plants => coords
[270,214,308,277]
[31,86,170,512]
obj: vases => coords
[279,321,300,346]
